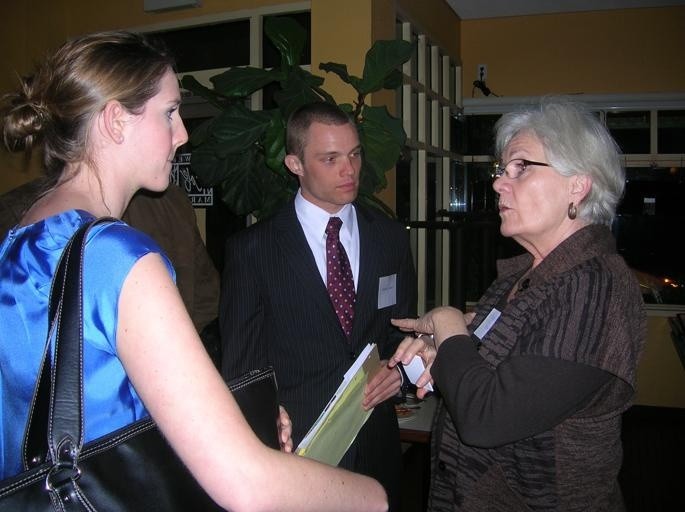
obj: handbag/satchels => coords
[1,367,284,512]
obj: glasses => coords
[489,157,550,178]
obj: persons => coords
[216,100,419,511]
[386,89,649,510]
[1,25,395,512]
[1,171,223,338]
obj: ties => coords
[325,217,356,340]
